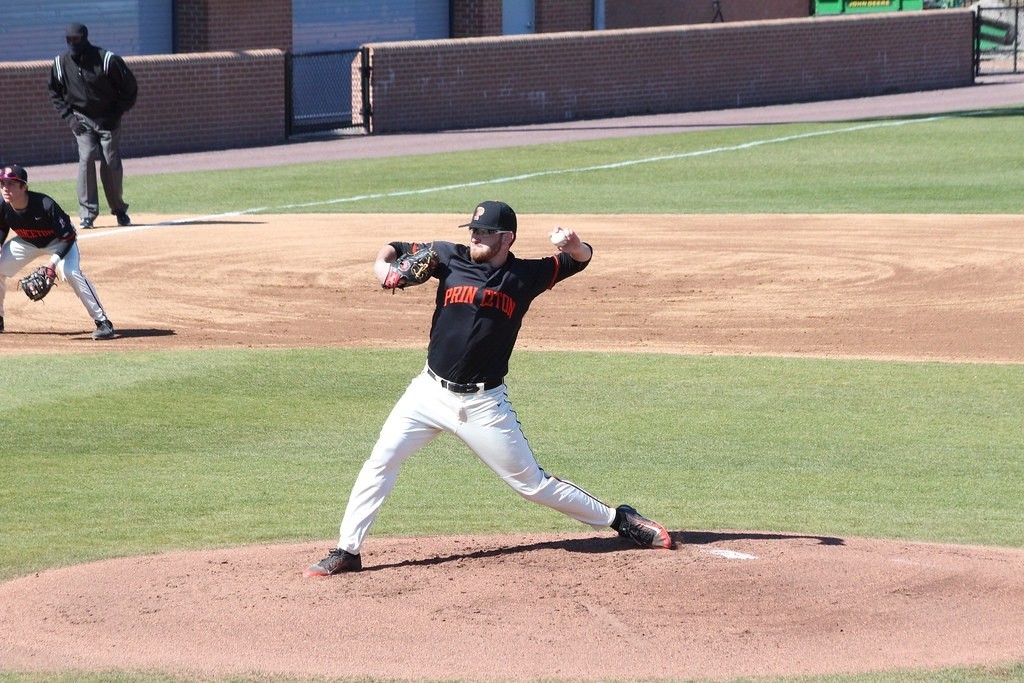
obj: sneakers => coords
[303,547,363,579]
[617,504,672,550]
[117,213,132,227]
[79,217,94,230]
[92,319,114,340]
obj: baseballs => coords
[551,230,567,246]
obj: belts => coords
[427,369,505,394]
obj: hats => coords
[458,200,517,236]
[65,23,86,36]
[0,164,27,184]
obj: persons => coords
[0,163,116,340]
[45,22,139,230]
[301,200,672,578]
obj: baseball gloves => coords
[16,266,59,305]
[382,247,439,295]
